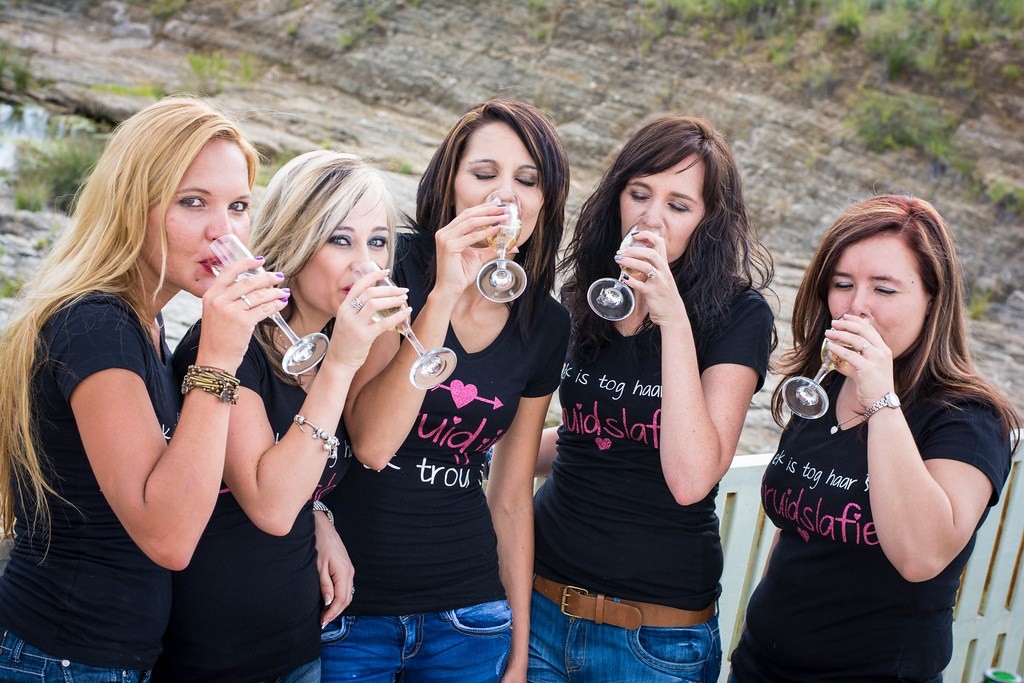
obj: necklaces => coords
[620,319,639,335]
[829,377,860,435]
[279,329,317,392]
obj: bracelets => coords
[293,414,340,469]
[313,500,334,526]
[180,364,240,405]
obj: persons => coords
[171,146,413,683]
[325,96,573,683]
[485,115,778,683]
[0,90,291,683]
[727,194,1020,683]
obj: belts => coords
[532,574,716,630]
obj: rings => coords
[352,298,362,310]
[648,269,656,278]
[241,294,253,310]
[860,341,870,352]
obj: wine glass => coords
[587,215,667,321]
[477,191,527,303]
[351,260,457,389]
[206,234,330,375]
[782,305,876,419]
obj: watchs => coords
[863,391,900,421]
